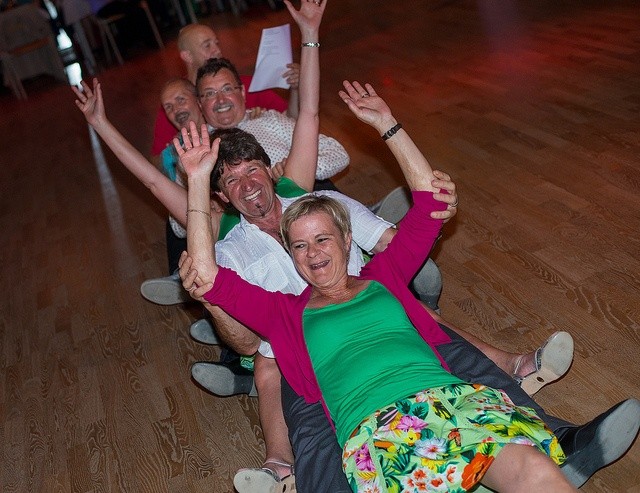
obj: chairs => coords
[0,0,164,101]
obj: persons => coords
[174,80,583,493]
[71,0,575,493]
[179,129,640,493]
[141,22,443,396]
[88,0,158,54]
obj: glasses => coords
[198,85,243,100]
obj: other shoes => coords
[140,268,196,305]
[191,358,259,396]
[367,185,412,225]
[189,318,221,344]
[412,258,443,316]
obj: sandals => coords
[232,460,297,493]
[512,331,575,397]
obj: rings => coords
[362,94,368,98]
[314,1,320,4]
[452,198,458,207]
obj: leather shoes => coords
[556,399,640,488]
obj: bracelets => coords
[187,210,211,216]
[301,43,320,47]
[382,124,401,140]
